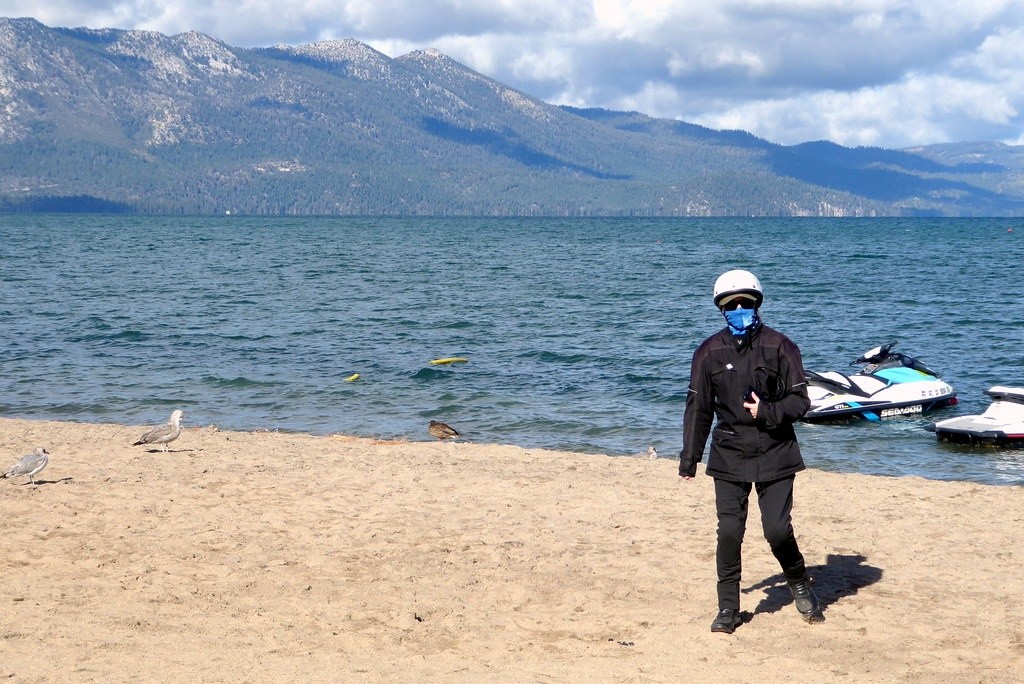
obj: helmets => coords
[713,270,764,306]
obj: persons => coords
[679,269,820,633]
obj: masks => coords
[722,307,757,335]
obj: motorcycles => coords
[921,384,1023,444]
[798,338,956,424]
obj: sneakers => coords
[711,609,742,634]
[790,576,821,615]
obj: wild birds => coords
[428,420,461,442]
[0,447,50,486]
[132,410,184,453]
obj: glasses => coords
[723,299,753,310]
